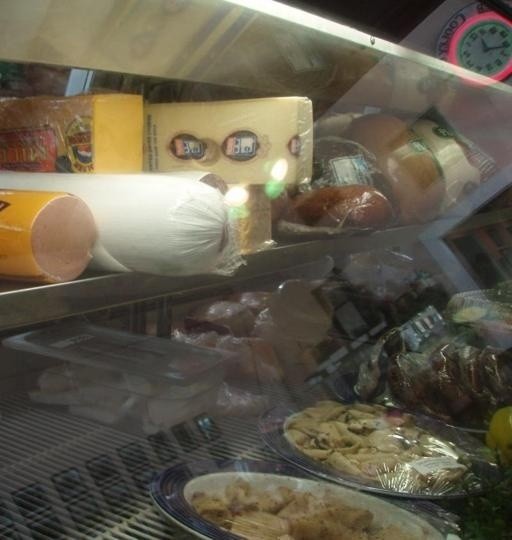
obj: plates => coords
[152,461,461,540]
[255,402,503,499]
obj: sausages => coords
[0,171,229,285]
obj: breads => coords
[284,186,391,229]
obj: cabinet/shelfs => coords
[0,0,512,540]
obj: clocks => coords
[450,13,512,85]
